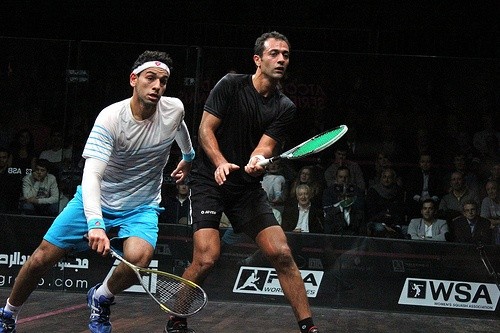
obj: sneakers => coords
[86,282,115,333]
[0,308,18,333]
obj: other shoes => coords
[164,314,195,333]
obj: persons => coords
[18,135,499,257]
[0,51,197,333]
[164,32,318,333]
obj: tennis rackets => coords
[255,123,349,168]
[84,232,208,317]
